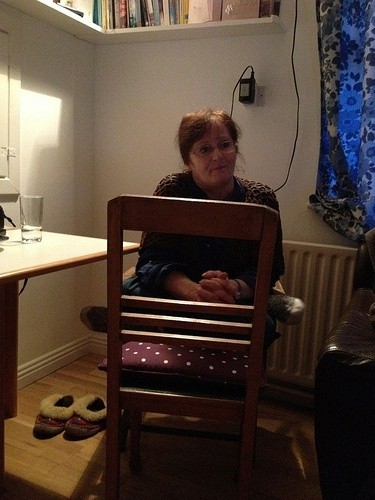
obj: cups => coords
[19,195,43,243]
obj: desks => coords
[0,226,143,500]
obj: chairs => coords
[103,195,279,500]
[313,223,375,500]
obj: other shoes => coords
[64,393,108,438]
[33,393,77,440]
[268,295,306,325]
[80,306,108,332]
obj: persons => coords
[80,108,304,349]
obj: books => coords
[92,0,273,29]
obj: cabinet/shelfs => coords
[0,0,286,46]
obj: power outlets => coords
[255,85,266,107]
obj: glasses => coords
[188,139,238,156]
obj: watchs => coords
[234,280,241,301]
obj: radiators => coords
[259,238,360,411]
